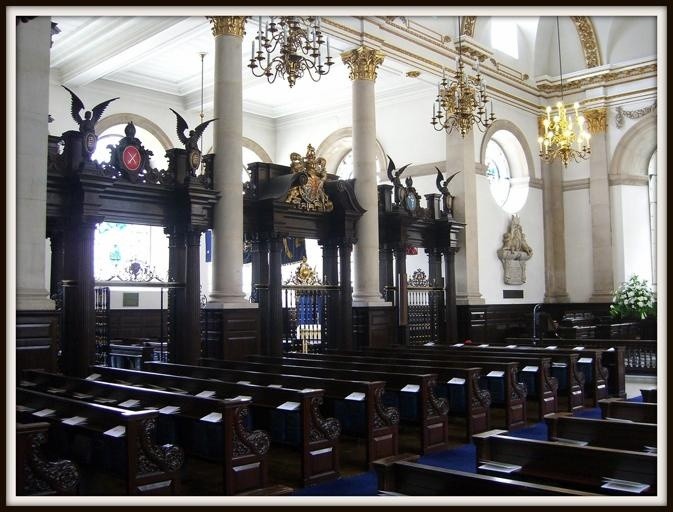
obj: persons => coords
[168,107,219,176]
[60,84,120,162]
[502,229,533,258]
[433,166,461,212]
[386,152,412,206]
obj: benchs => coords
[16,318,657,497]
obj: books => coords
[47,388,66,394]
[236,381,251,384]
[20,381,37,387]
[225,395,252,402]
[422,341,615,352]
[477,456,523,474]
[599,474,651,493]
[550,436,589,447]
[551,361,568,368]
[200,411,223,423]
[74,392,181,415]
[16,406,35,412]
[277,401,300,411]
[577,356,593,363]
[117,379,215,398]
[399,383,420,393]
[62,416,88,427]
[485,370,505,378]
[521,364,539,372]
[643,443,657,455]
[345,392,366,401]
[104,425,125,438]
[446,376,466,386]
[267,384,282,388]
[86,373,102,381]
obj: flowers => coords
[609,273,657,321]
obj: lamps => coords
[536,17,593,167]
[430,16,497,141]
[247,16,335,90]
